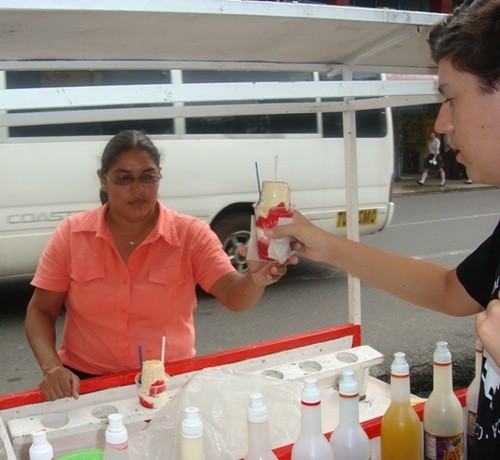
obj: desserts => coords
[140,359,170,409]
[254,180,295,265]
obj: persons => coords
[416,129,446,186]
[265,0,500,460]
[25,131,300,403]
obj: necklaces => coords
[130,240,135,246]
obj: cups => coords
[134,373,172,408]
[253,203,296,260]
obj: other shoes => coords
[416,179,425,186]
[440,181,447,186]
[464,180,472,184]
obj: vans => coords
[0,68,395,283]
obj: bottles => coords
[28,428,55,460]
[465,338,483,460]
[180,406,204,460]
[423,341,465,460]
[103,413,131,460]
[290,378,334,460]
[243,393,279,460]
[380,352,422,460]
[329,370,371,460]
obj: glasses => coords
[107,172,162,185]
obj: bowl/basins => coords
[56,449,105,460]
